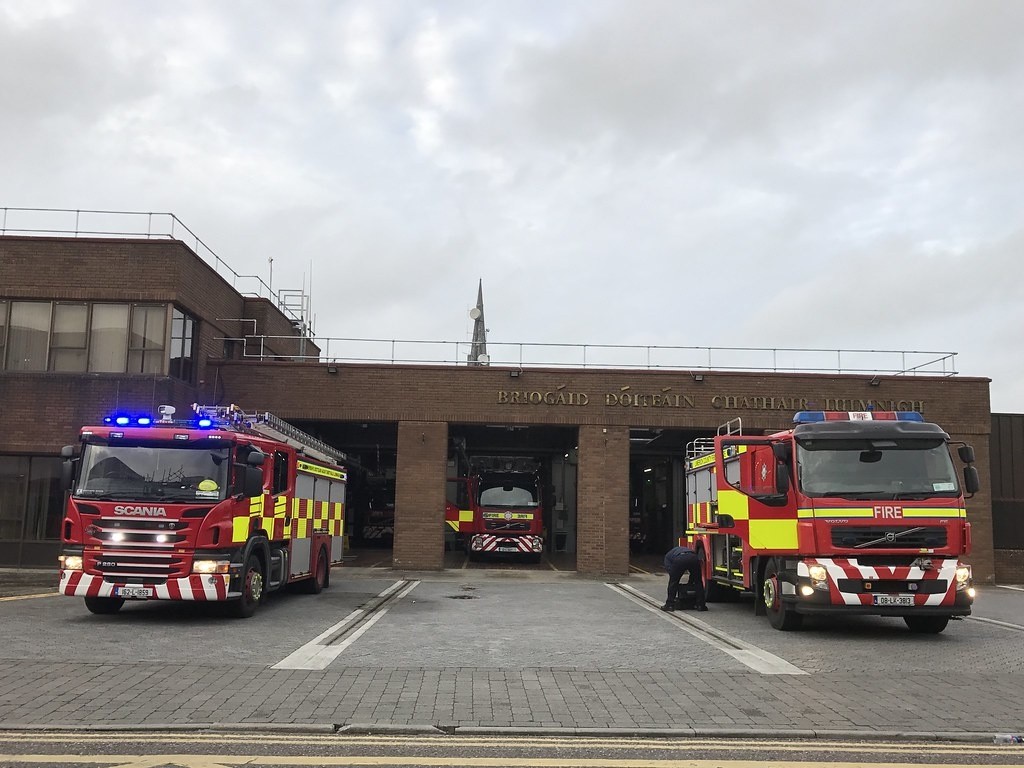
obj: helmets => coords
[198,479,219,491]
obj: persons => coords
[661,547,708,612]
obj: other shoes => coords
[661,604,674,611]
[698,605,708,611]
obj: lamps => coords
[695,374,703,381]
[510,371,519,377]
[328,366,337,373]
[872,378,880,385]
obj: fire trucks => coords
[679,409,979,633]
[57,364,348,618]
[445,456,550,562]
